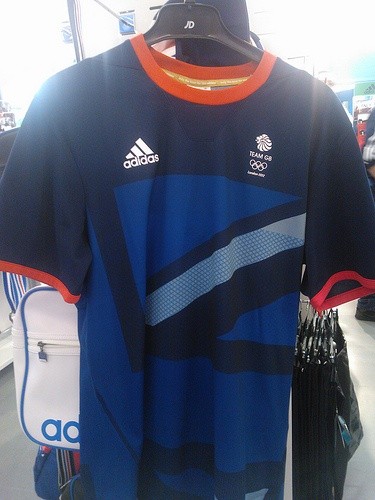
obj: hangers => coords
[295,299,338,365]
[143,0,263,62]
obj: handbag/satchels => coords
[3,272,79,452]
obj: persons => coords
[355,106,375,321]
